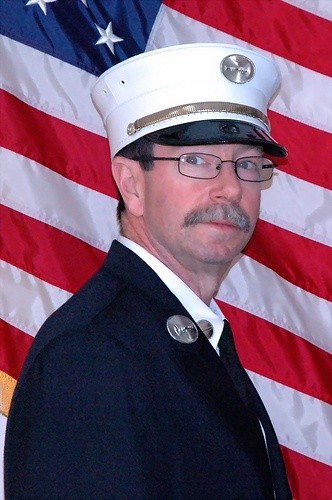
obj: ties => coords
[215,318,252,419]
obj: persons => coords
[4,42,292,500]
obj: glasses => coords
[131,152,278,182]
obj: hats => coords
[89,42,288,159]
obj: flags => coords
[0,0,332,500]
